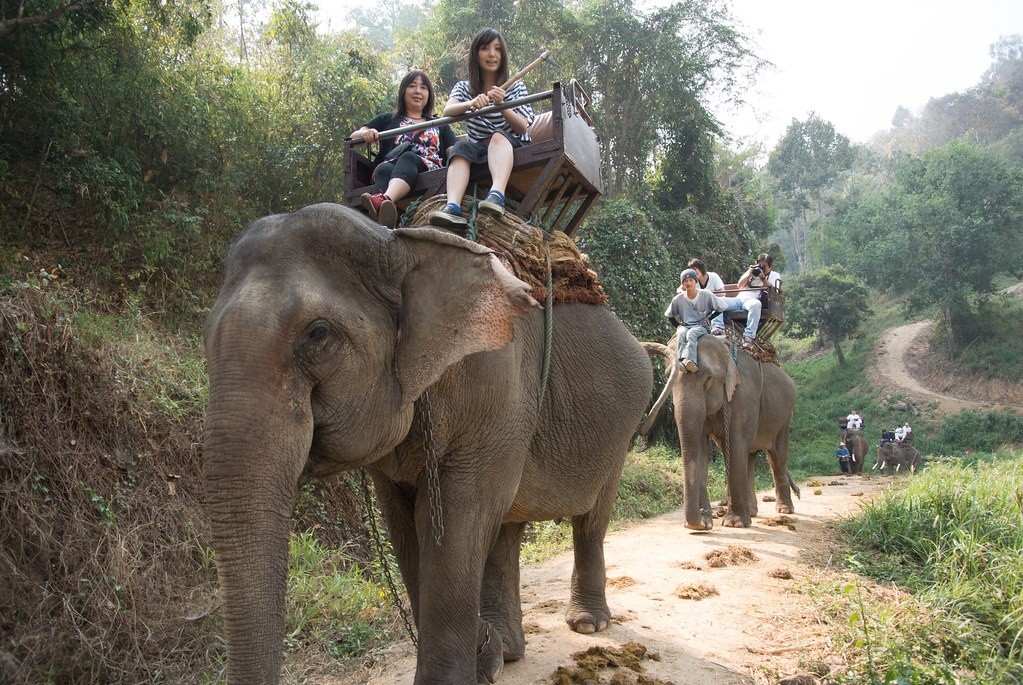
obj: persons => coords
[889,422,911,443]
[677,258,726,297]
[429,28,534,229]
[846,410,862,430]
[710,253,781,348]
[877,429,891,449]
[349,71,457,230]
[836,441,850,474]
[664,269,729,374]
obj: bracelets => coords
[760,276,765,281]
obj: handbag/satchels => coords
[385,142,411,160]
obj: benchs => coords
[345,78,602,241]
[839,416,866,428]
[711,279,784,350]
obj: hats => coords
[839,442,846,446]
[680,269,697,284]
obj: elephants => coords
[661,330,801,534]
[197,203,678,685]
[872,441,921,477]
[843,428,869,472]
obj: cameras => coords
[751,264,761,277]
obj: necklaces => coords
[406,116,424,120]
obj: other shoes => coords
[685,360,699,373]
[742,337,752,348]
[711,328,722,335]
[680,362,689,374]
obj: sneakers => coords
[429,206,468,230]
[362,188,389,217]
[378,199,397,229]
[478,193,505,215]
